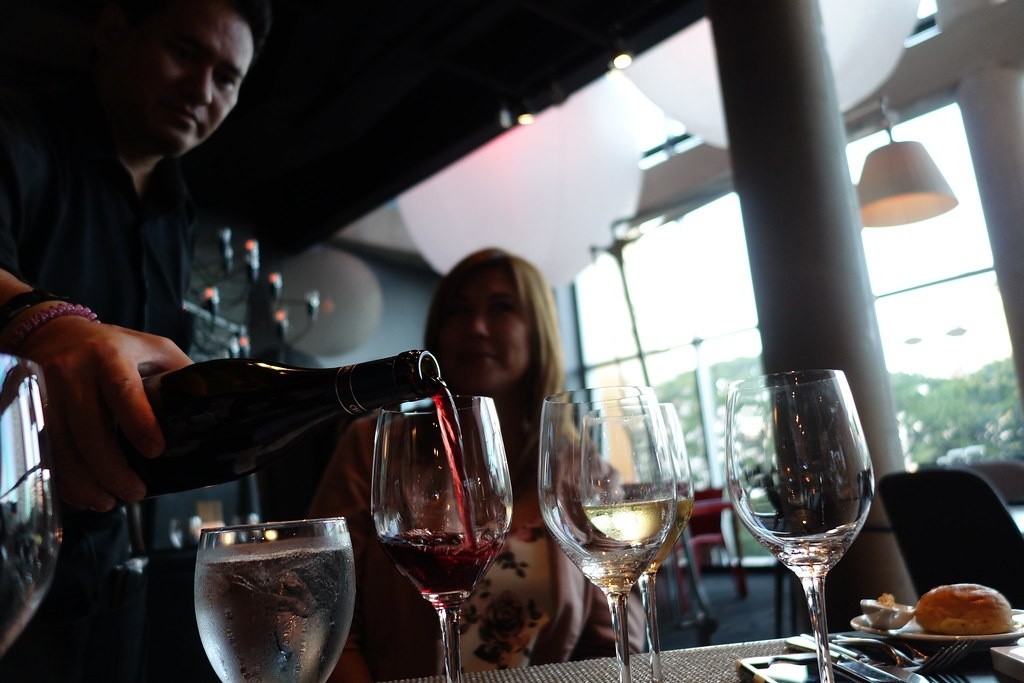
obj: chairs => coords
[876,466,1024,610]
[655,488,722,621]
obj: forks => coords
[831,635,977,672]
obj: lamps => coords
[856,96,959,228]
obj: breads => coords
[915,583,1014,635]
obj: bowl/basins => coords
[860,599,916,630]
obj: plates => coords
[850,609,1024,651]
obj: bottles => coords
[39,350,441,517]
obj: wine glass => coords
[371,396,514,683]
[538,387,689,683]
[726,369,875,683]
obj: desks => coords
[378,633,808,683]
[691,496,749,598]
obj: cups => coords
[0,353,60,654]
[194,517,356,683]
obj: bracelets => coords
[0,289,71,330]
[0,304,101,357]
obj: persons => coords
[0,1,272,683]
[300,250,648,683]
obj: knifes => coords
[785,633,931,683]
